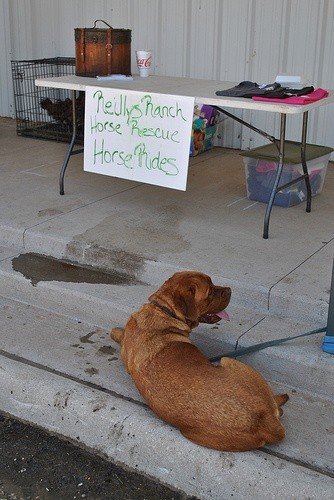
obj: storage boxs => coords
[11,58,84,145]
[238,139,333,208]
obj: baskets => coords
[12,57,88,144]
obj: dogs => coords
[109,269,290,452]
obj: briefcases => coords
[74,20,133,76]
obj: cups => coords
[135,50,153,77]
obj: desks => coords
[35,74,334,239]
